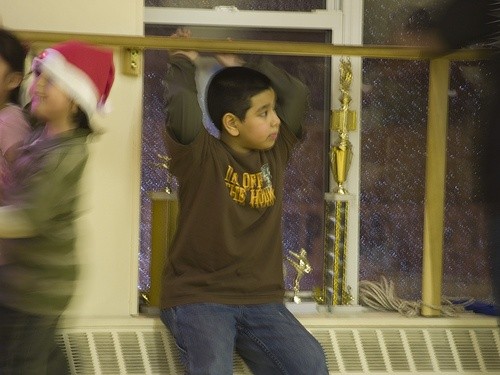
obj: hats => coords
[32,38,116,136]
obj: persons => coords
[0,27,33,202]
[393,0,500,310]
[163,29,331,375]
[0,39,113,375]
